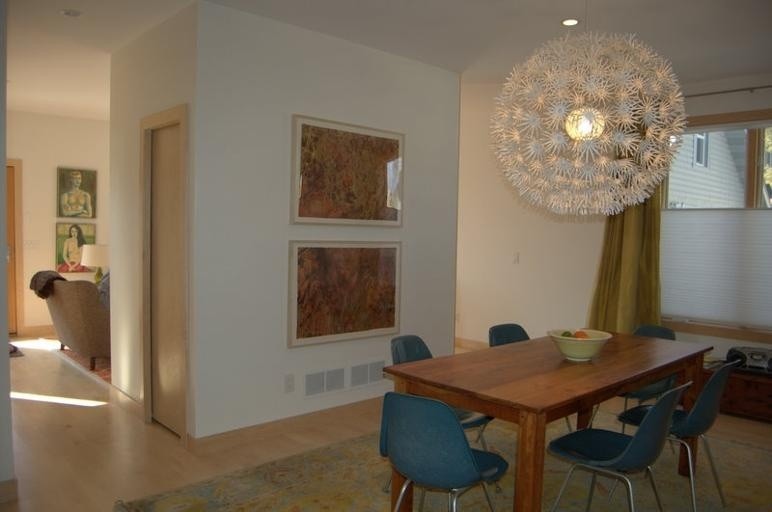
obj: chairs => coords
[383,335,504,494]
[379,391,509,511]
[474,324,572,444]
[46,280,110,370]
[607,359,741,512]
[614,324,675,435]
[547,381,697,511]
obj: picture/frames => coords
[55,222,98,274]
[56,167,98,219]
[286,240,402,348]
[288,114,406,228]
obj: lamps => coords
[80,244,108,284]
[488,1,690,216]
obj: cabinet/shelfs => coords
[676,366,772,425]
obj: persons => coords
[61,169,92,217]
[59,223,86,271]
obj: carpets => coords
[111,405,771,512]
[60,349,111,386]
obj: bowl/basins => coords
[545,328,612,363]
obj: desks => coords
[383,328,715,512]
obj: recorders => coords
[726,345,772,374]
